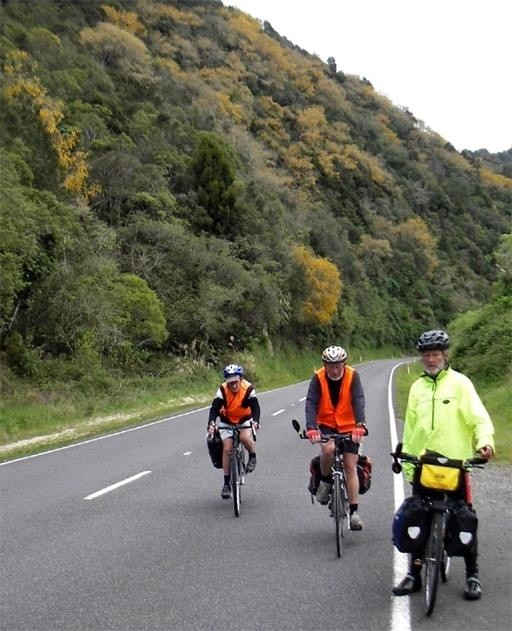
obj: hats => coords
[224,375,240,383]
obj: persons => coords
[205,364,260,500]
[306,345,368,532]
[392,330,496,602]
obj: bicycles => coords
[386,439,491,619]
[297,431,353,557]
[207,420,262,518]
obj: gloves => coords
[306,427,321,445]
[351,426,367,444]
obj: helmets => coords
[415,329,450,352]
[320,345,348,364]
[223,364,244,379]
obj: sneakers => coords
[315,480,331,504]
[391,571,423,596]
[245,457,257,474]
[463,568,482,601]
[349,511,363,531]
[220,483,232,500]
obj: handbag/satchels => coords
[413,448,466,496]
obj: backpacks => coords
[445,500,479,558]
[307,455,323,504]
[356,454,373,496]
[206,426,224,470]
[391,495,436,555]
